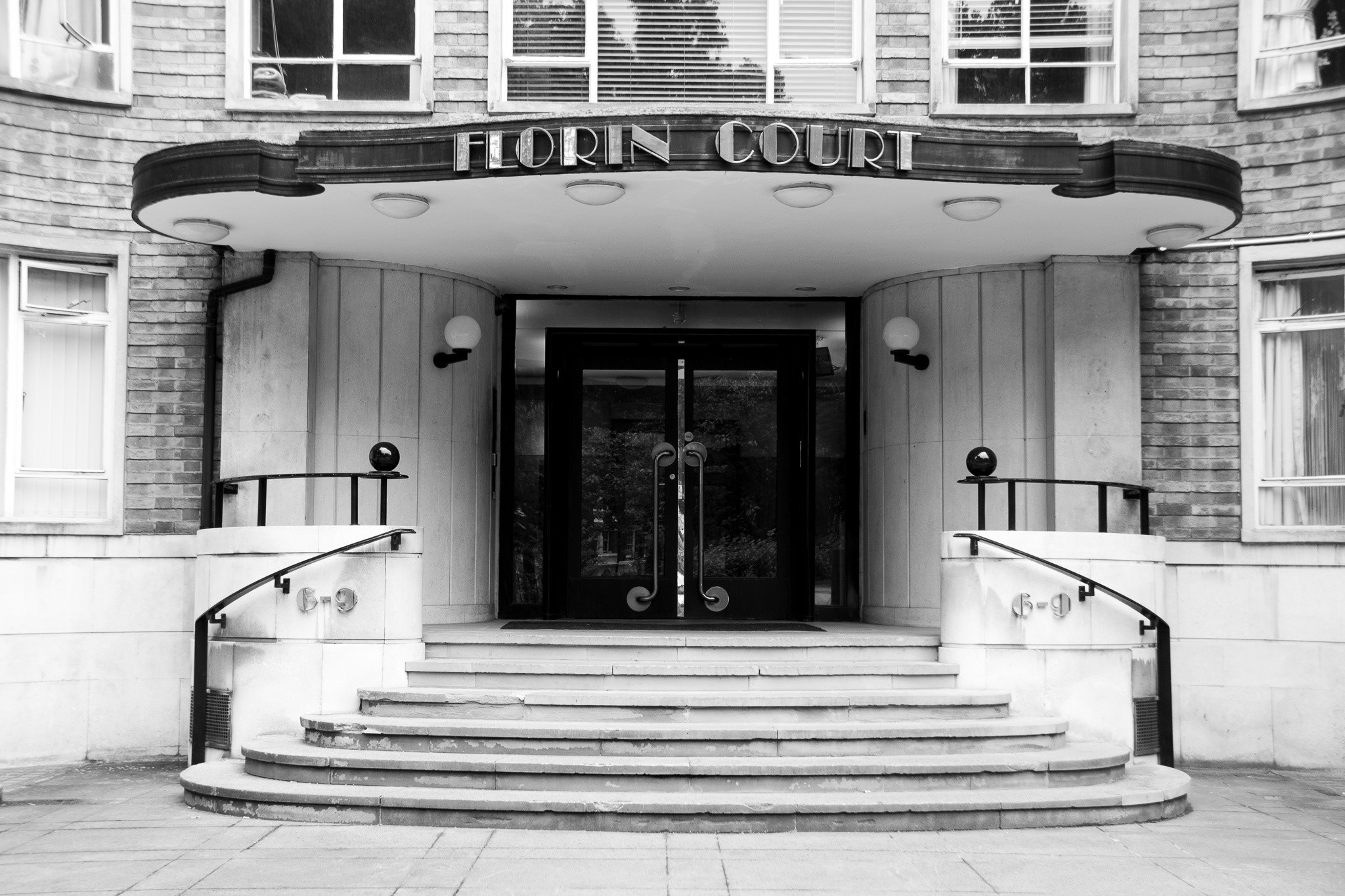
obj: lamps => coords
[773,183,833,210]
[372,196,429,220]
[1146,227,1206,253]
[434,317,480,369]
[884,314,929,374]
[943,199,1001,223]
[172,221,229,245]
[564,181,626,207]
